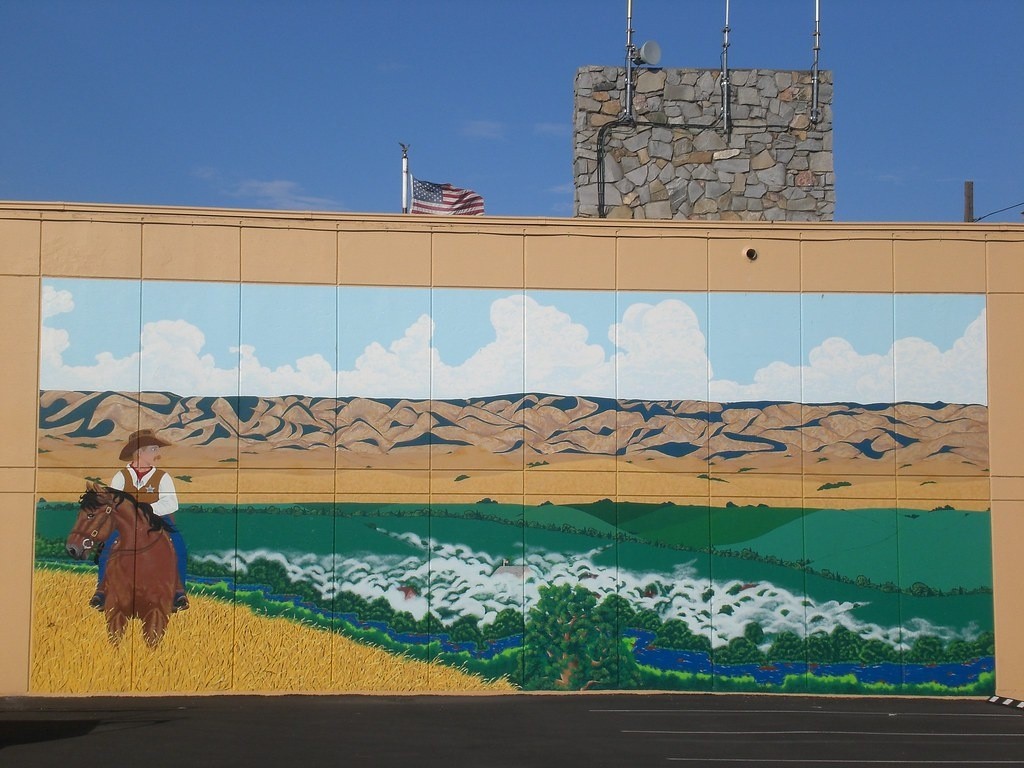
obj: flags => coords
[410,174,484,216]
